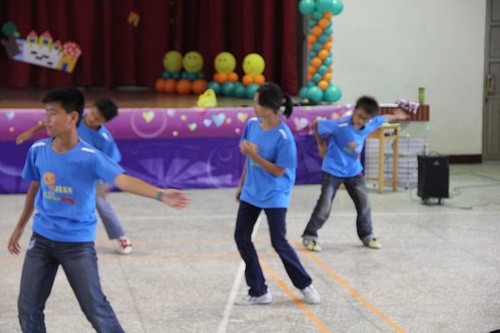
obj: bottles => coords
[419,87,425,104]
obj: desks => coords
[363,122,400,193]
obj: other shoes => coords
[233,288,272,305]
[301,284,320,304]
[303,238,321,251]
[366,238,381,248]
[115,236,132,254]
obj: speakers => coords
[417,155,450,198]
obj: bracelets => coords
[155,189,163,202]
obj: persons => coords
[16,98,132,255]
[7,87,189,333]
[302,97,410,251]
[234,82,321,304]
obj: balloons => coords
[298,0,344,102]
[155,51,266,97]
